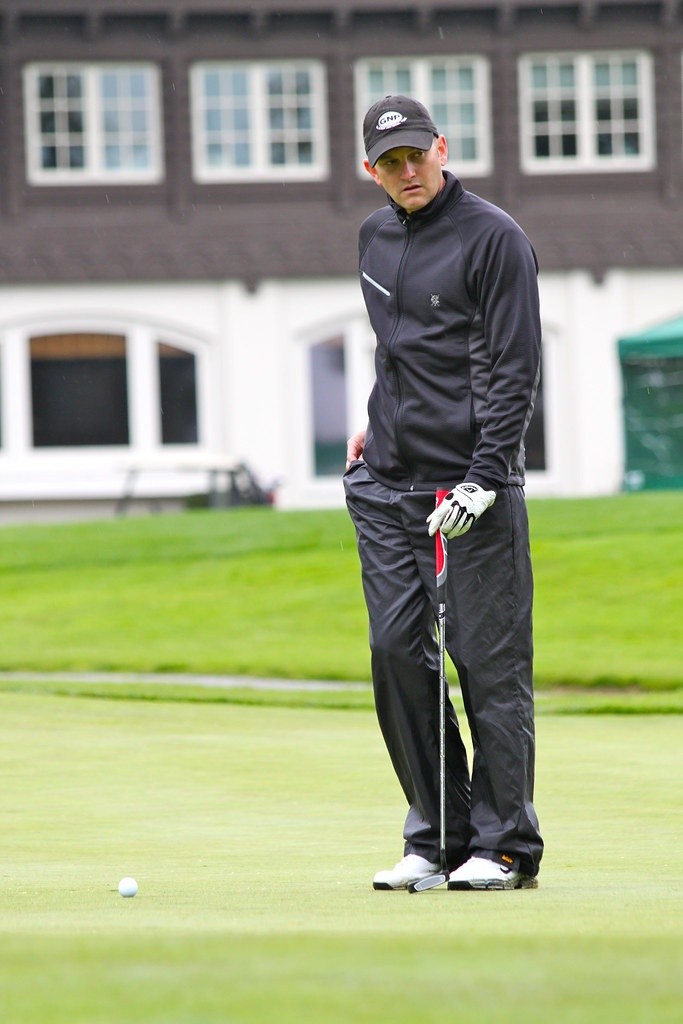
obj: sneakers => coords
[373,854,441,890]
[448,857,538,889]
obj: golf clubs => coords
[404,486,450,893]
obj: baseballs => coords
[118,878,138,897]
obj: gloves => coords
[426,483,496,539]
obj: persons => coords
[341,95,546,894]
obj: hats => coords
[364,95,438,168]
